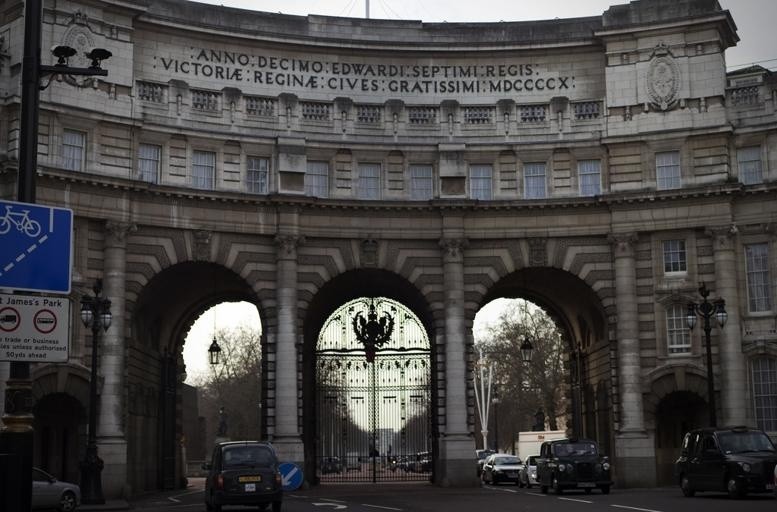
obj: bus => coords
[200,437,232,476]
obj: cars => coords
[322,451,434,475]
[476,450,540,490]
[30,465,81,511]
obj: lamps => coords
[520,300,533,362]
[208,305,221,364]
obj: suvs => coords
[535,439,615,494]
[677,428,776,498]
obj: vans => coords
[201,440,283,512]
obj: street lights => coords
[0,42,114,511]
[686,280,728,428]
[491,392,499,454]
[78,276,114,507]
